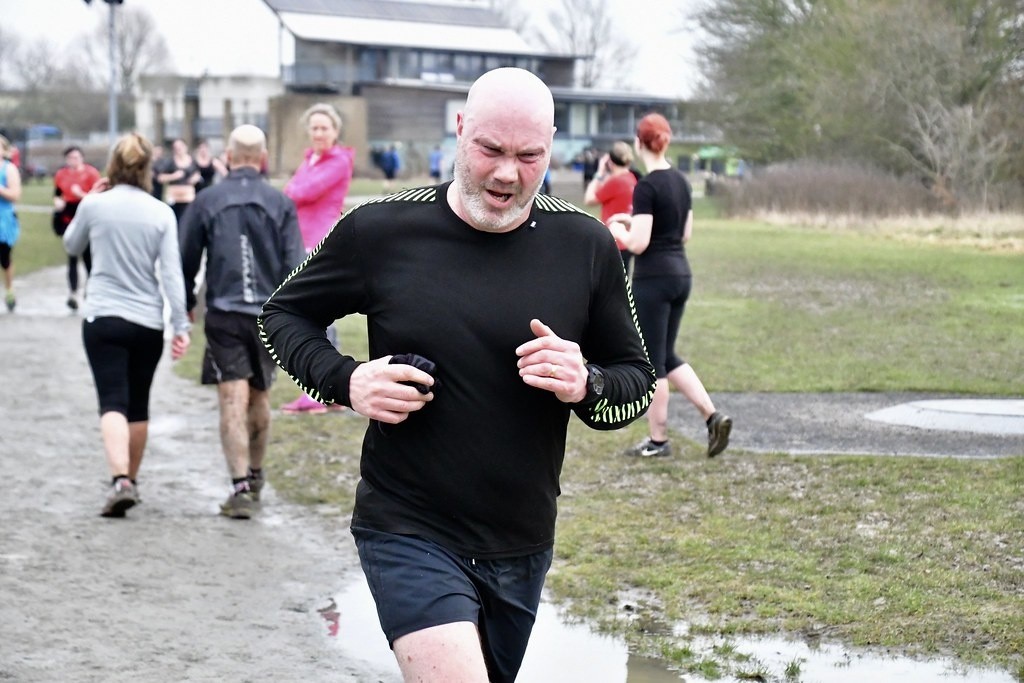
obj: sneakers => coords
[134,483,143,504]
[620,436,673,458]
[707,411,732,458]
[325,401,349,410]
[100,477,137,520]
[249,476,264,499]
[219,492,253,519]
[283,392,328,415]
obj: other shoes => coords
[5,289,16,312]
[67,297,78,309]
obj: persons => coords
[604,113,732,457]
[256,66,657,683]
[61,133,191,517]
[177,124,311,518]
[51,142,103,309]
[146,139,228,222]
[367,140,403,194]
[427,143,445,181]
[538,169,553,195]
[0,134,22,311]
[279,103,353,413]
[582,141,642,275]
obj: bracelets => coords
[594,174,603,180]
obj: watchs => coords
[576,364,605,407]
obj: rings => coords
[548,364,557,377]
